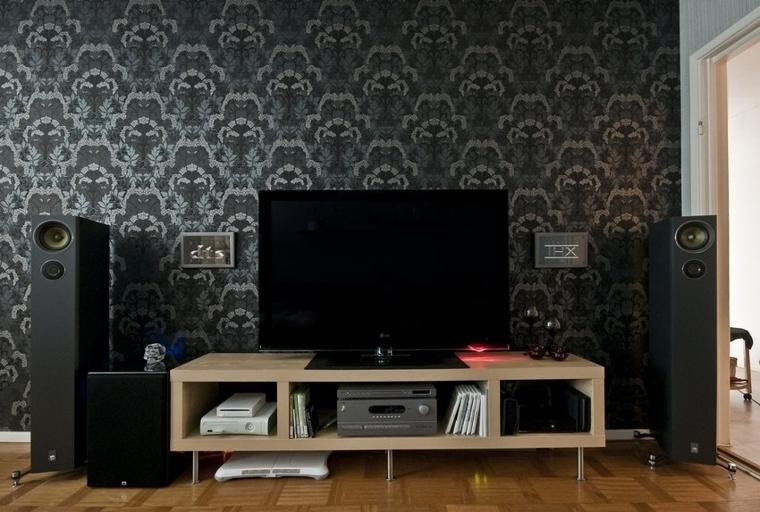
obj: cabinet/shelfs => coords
[167,352,607,481]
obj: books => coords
[442,384,487,438]
[289,390,318,439]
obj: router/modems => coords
[216,391,267,419]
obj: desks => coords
[730,327,753,393]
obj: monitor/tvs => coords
[257,189,511,372]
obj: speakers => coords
[30,212,110,474]
[88,376,191,488]
[645,214,719,466]
